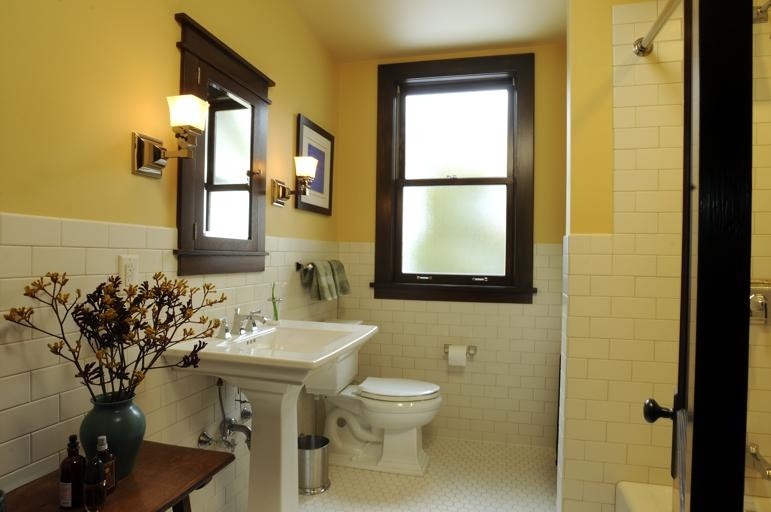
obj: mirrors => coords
[203,80,252,240]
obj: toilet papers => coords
[447,344,469,368]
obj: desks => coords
[0,441,234,512]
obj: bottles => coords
[95,435,117,495]
[59,433,86,512]
[84,444,107,512]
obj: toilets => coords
[304,319,443,479]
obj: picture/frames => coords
[294,114,336,220]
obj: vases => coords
[75,392,146,476]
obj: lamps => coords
[131,91,205,179]
[269,153,319,205]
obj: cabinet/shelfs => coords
[194,59,261,252]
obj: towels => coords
[328,260,352,297]
[302,260,336,302]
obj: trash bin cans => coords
[298,435,331,496]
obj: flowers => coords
[12,260,213,398]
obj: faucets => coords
[229,307,269,336]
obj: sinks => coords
[160,319,380,386]
